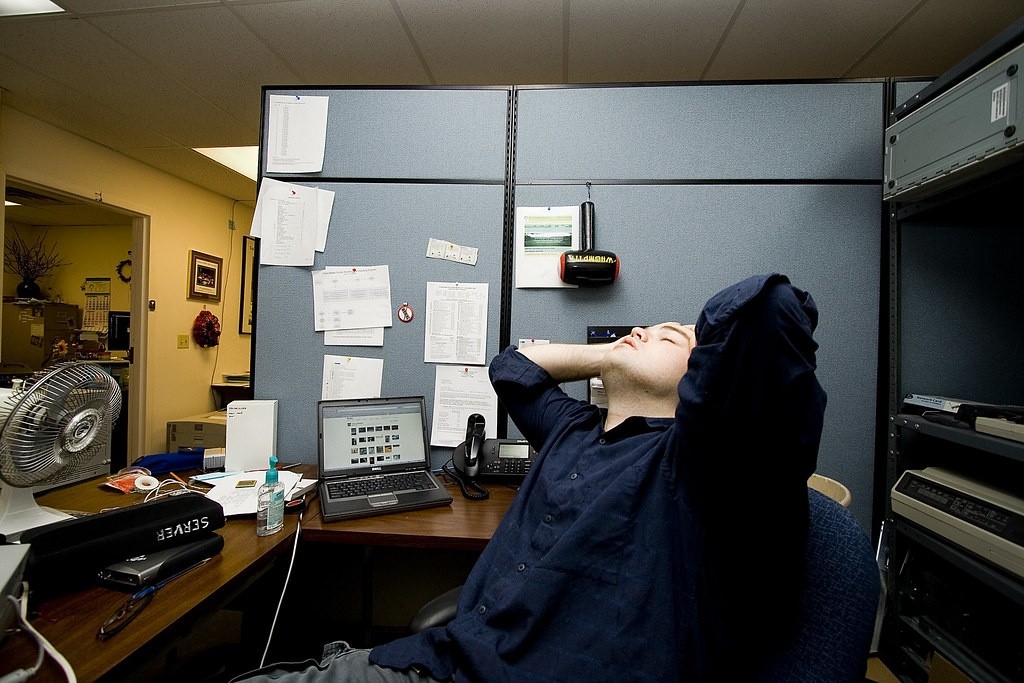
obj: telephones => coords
[451,412,538,481]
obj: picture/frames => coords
[186,248,224,303]
[239,235,255,335]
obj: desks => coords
[210,382,250,410]
[0,460,863,683]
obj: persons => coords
[201,273,826,683]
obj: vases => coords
[50,355,62,366]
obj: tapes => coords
[134,475,159,493]
[285,499,307,515]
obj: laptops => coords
[318,395,454,524]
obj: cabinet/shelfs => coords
[865,14,1024,683]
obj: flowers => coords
[52,338,69,357]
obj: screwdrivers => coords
[275,463,301,470]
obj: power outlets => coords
[177,334,190,350]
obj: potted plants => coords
[3,222,75,298]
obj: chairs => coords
[408,467,883,683]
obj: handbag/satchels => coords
[131,447,204,475]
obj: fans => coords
[0,355,124,542]
